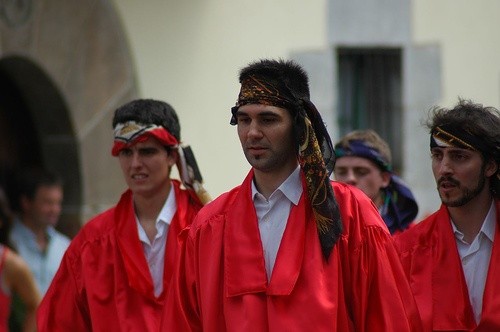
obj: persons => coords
[38,98,217,332]
[162,58,420,332]
[1,163,73,332]
[392,95,500,332]
